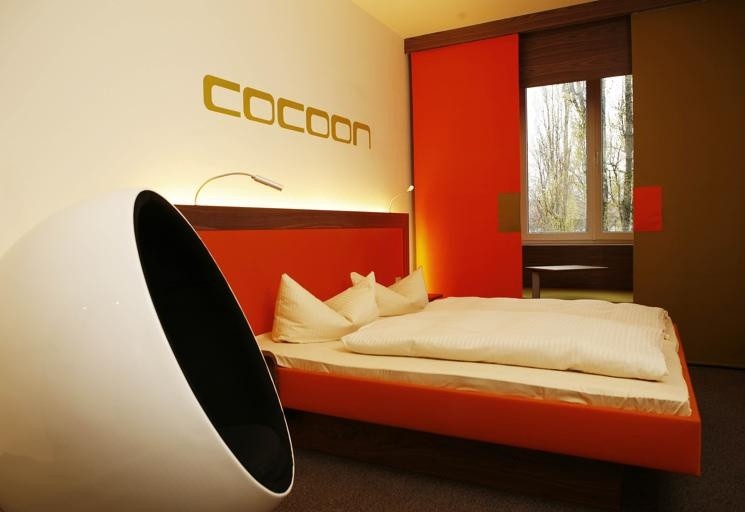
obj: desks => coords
[524,264,609,298]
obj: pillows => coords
[271,266,430,345]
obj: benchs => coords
[173,204,705,511]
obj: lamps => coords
[193,169,283,207]
